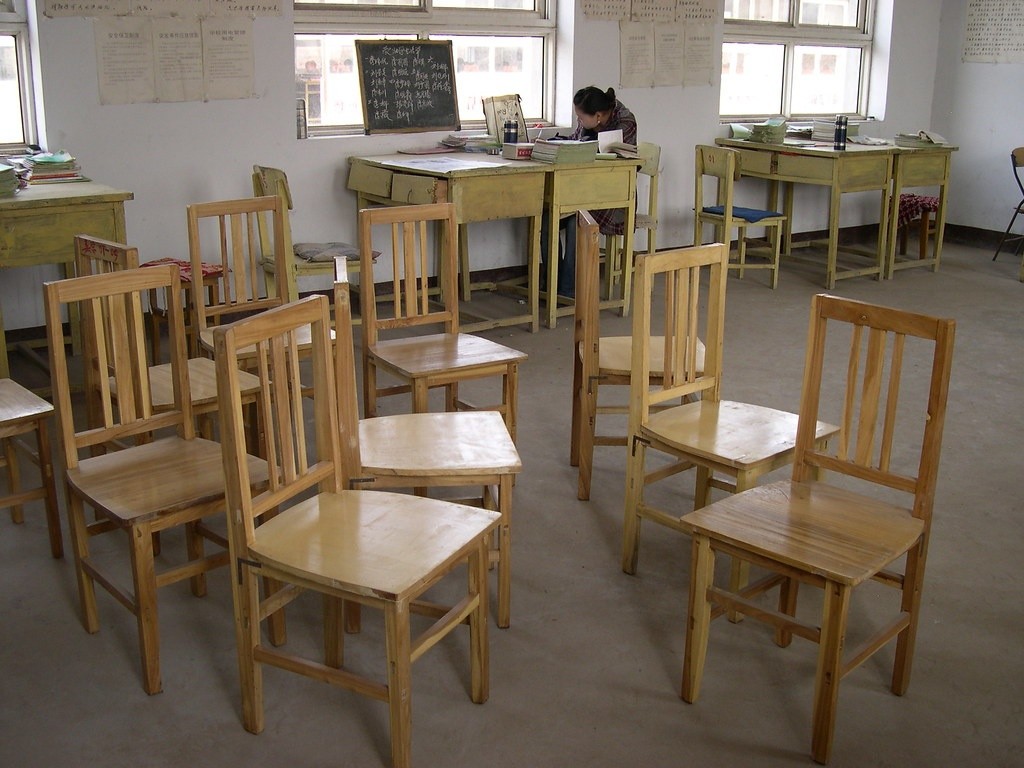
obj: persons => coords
[531,86,642,308]
[305,59,352,75]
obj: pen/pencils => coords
[535,128,543,146]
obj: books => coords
[730,118,951,146]
[0,149,93,199]
[397,134,644,164]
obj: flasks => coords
[504,121,518,143]
[834,115,848,150]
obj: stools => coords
[887,193,940,260]
[140,258,233,366]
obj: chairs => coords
[251,164,382,343]
[677,293,957,766]
[0,378,65,560]
[599,142,661,302]
[570,209,705,501]
[358,203,528,498]
[185,195,337,455]
[623,242,841,624]
[992,147,1024,261]
[73,233,275,557]
[333,255,523,635]
[693,144,787,291]
[212,294,502,768]
[42,263,287,697]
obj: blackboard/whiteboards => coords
[355,39,460,135]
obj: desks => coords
[796,138,959,280]
[715,138,900,291]
[0,181,134,400]
[347,150,554,334]
[482,152,645,330]
